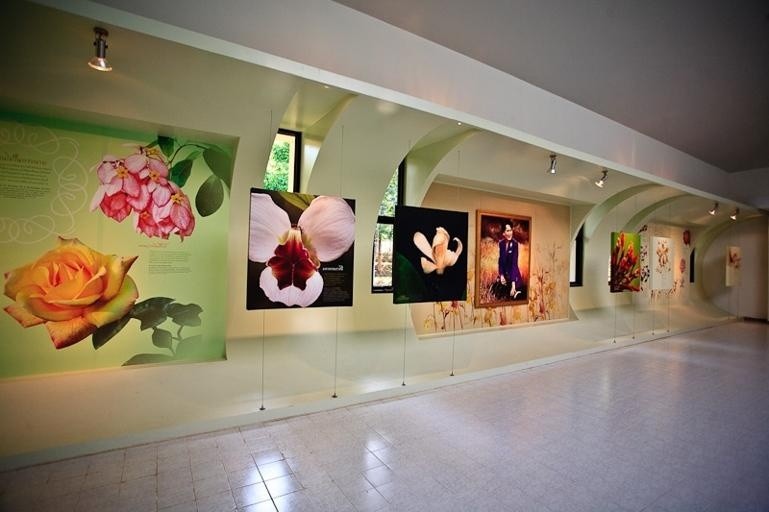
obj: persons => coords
[487,221,528,299]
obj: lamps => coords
[89,27,112,72]
[545,154,608,188]
[708,203,740,221]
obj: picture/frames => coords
[475,208,532,307]
